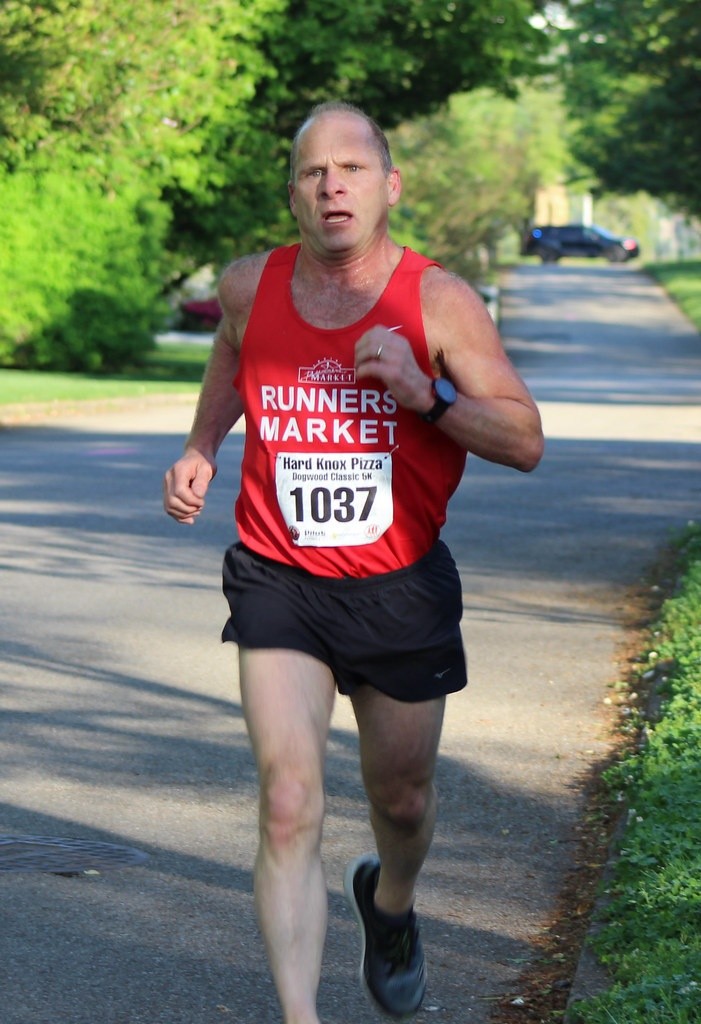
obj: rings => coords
[376,344,384,358]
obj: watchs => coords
[421,376,458,426]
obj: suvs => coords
[520,224,640,267]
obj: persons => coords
[161,102,545,1024]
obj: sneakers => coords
[344,854,429,1023]
